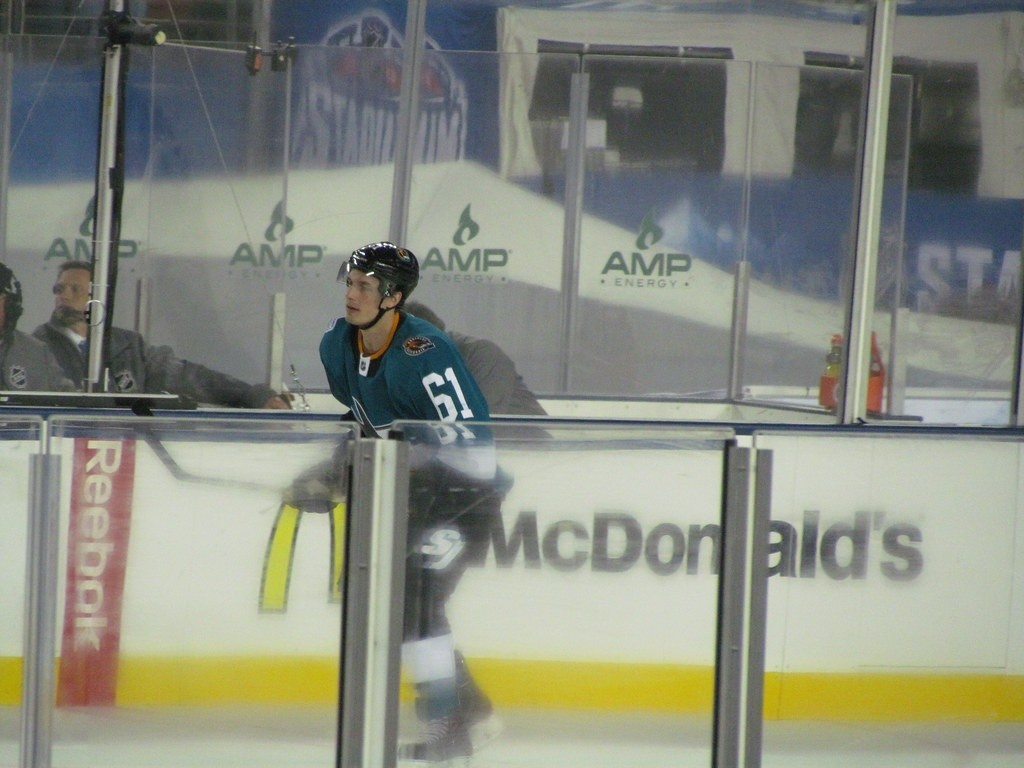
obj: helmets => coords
[349,241,419,303]
[0,261,22,330]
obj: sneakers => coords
[395,712,473,768]
[458,689,502,751]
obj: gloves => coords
[284,460,341,514]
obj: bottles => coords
[866,331,885,411]
[819,335,843,407]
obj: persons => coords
[30,261,291,408]
[284,241,516,768]
[401,300,552,417]
[0,261,76,394]
